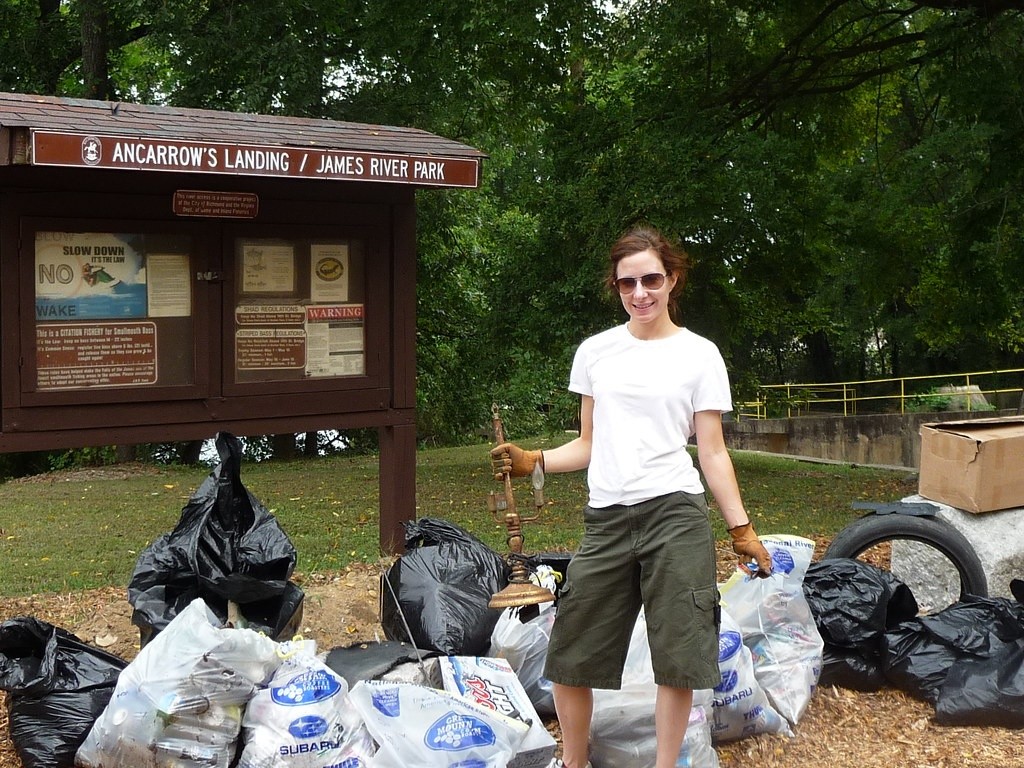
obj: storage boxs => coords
[918,415,1024,515]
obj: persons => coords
[490,230,772,768]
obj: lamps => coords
[487,401,557,608]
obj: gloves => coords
[727,521,772,579]
[491,443,545,481]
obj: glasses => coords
[613,274,672,294]
